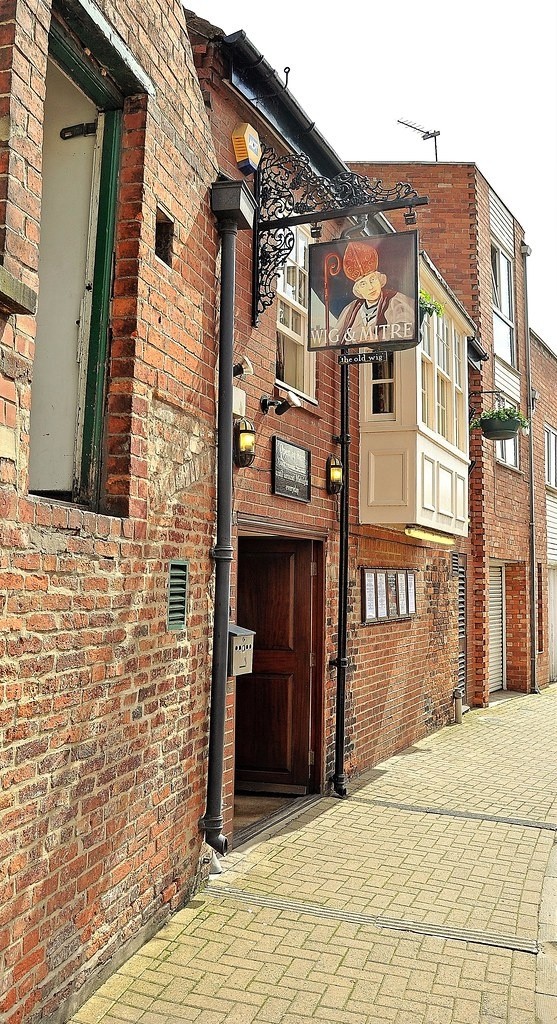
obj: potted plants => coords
[470,407,532,440]
[420,288,447,329]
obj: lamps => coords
[260,391,301,416]
[405,525,456,545]
[326,453,344,495]
[232,355,253,378]
[233,420,256,468]
